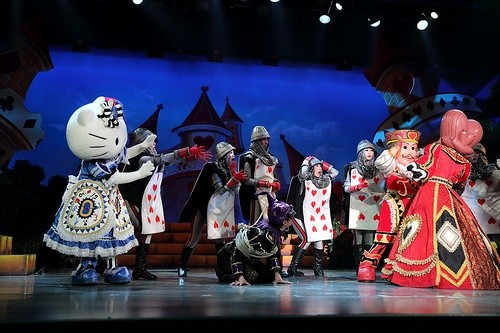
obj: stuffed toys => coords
[43,98,157,284]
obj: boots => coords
[178,245,195,277]
[287,246,306,276]
[132,243,158,280]
[313,247,324,276]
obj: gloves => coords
[226,169,247,189]
[258,180,281,192]
[174,144,206,160]
[349,182,369,193]
[187,151,213,163]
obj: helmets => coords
[216,142,237,160]
[251,125,270,141]
[133,128,154,145]
[473,143,486,156]
[306,156,324,172]
[356,139,376,153]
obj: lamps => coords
[415,6,440,33]
[367,13,383,31]
[318,0,345,26]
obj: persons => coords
[216,192,296,286]
[382,109,500,289]
[344,140,384,273]
[357,130,421,281]
[239,126,280,225]
[460,142,500,255]
[121,129,212,280]
[286,156,339,276]
[177,142,248,277]
